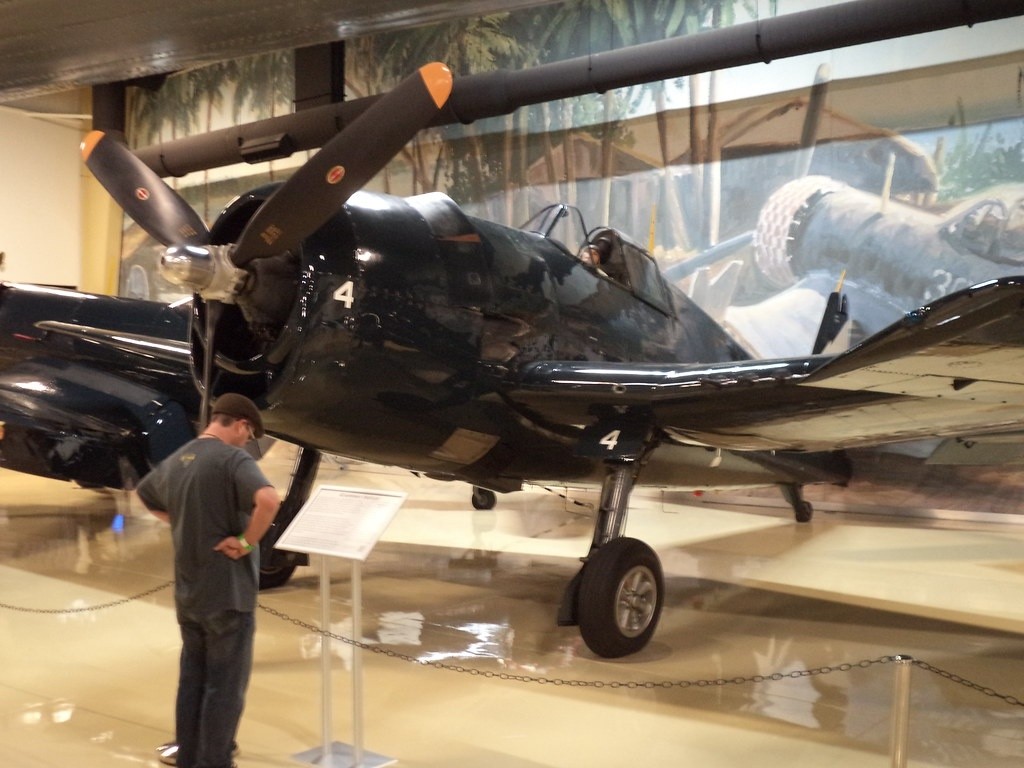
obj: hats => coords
[212,392,264,439]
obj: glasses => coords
[248,426,256,441]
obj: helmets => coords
[580,245,602,255]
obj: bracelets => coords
[238,534,255,550]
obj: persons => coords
[577,244,609,278]
[136,393,281,768]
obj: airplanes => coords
[34,59,1024,662]
[658,62,1024,359]
[0,279,280,491]
